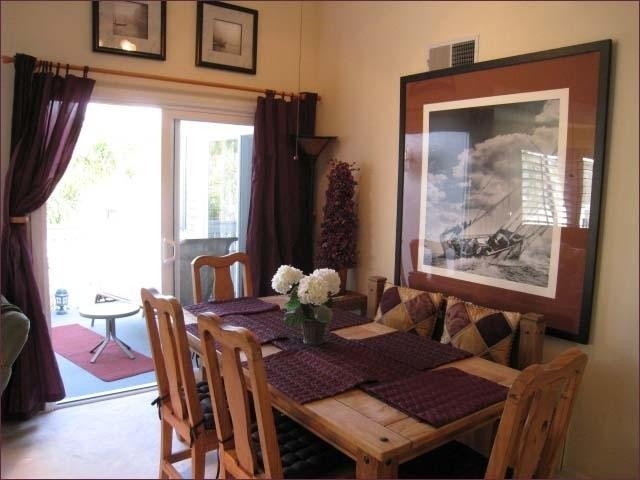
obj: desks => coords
[79,296,141,364]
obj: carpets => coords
[49,322,155,382]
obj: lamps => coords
[289,134,338,276]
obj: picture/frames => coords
[394,37,612,345]
[91,0,259,74]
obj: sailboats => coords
[439,150,558,262]
